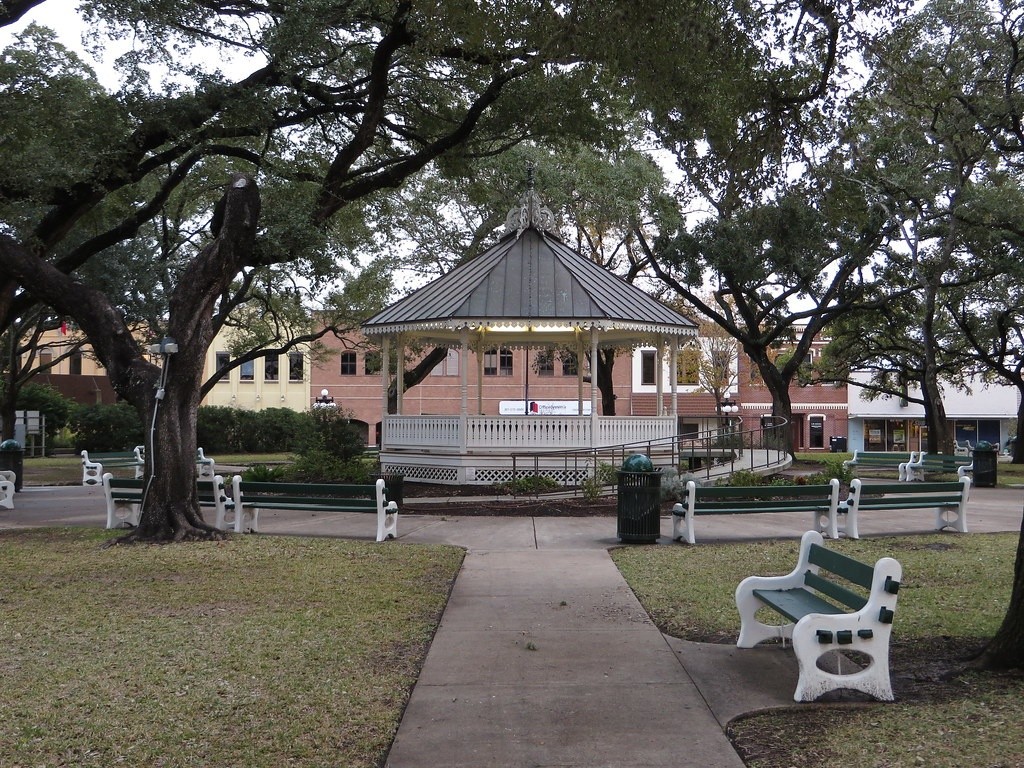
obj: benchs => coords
[195,448,216,477]
[231,474,400,543]
[669,478,840,544]
[844,451,920,482]
[102,473,251,533]
[80,448,145,486]
[952,439,1001,456]
[905,452,977,483]
[733,531,905,704]
[818,475,971,539]
[0,469,17,509]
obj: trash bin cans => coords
[370,472,407,506]
[616,454,665,543]
[0,439,24,494]
[830,435,848,453]
[970,440,999,488]
